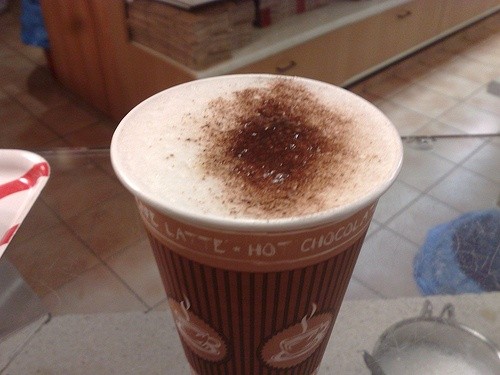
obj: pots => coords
[364,300,499,374]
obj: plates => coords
[0,147,51,262]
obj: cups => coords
[108,74,403,374]
